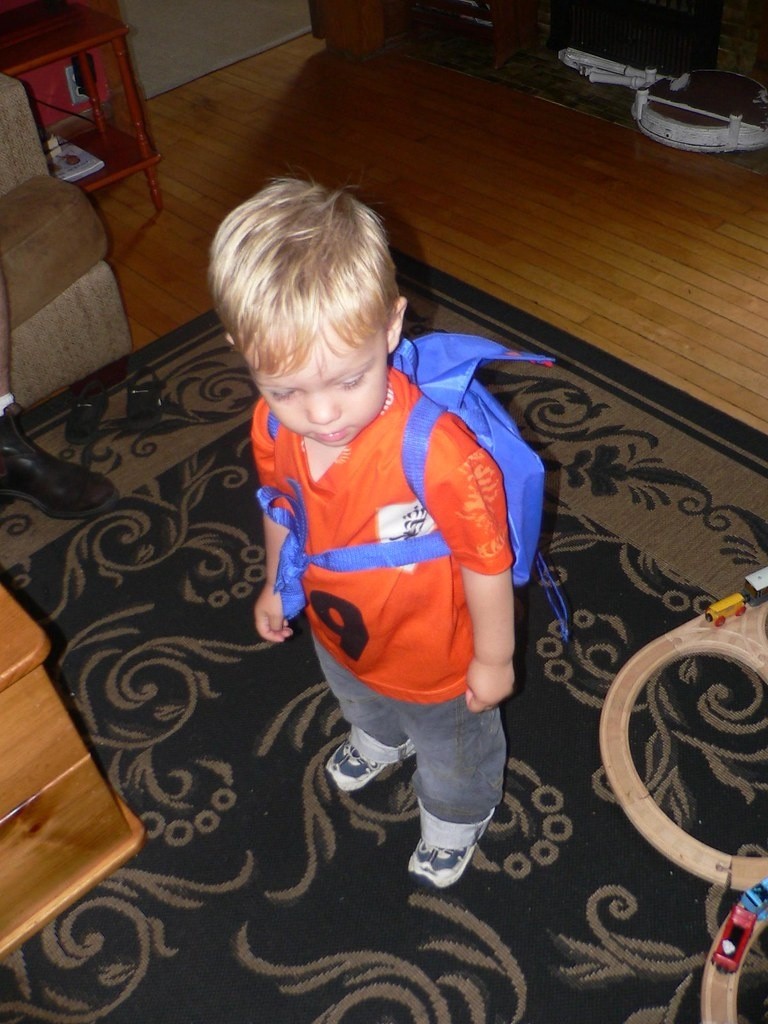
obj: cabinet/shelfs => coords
[382,0,540,70]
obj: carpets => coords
[0,249,768,1024]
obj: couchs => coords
[0,73,131,409]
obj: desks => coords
[0,585,147,962]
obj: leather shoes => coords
[0,403,119,517]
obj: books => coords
[40,133,105,182]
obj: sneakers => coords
[405,837,479,890]
[325,739,389,792]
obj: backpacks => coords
[266,331,555,591]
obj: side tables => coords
[0,2,165,213]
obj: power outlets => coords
[64,66,91,105]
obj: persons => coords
[206,178,516,892]
[0,270,119,519]
[66,155,79,165]
